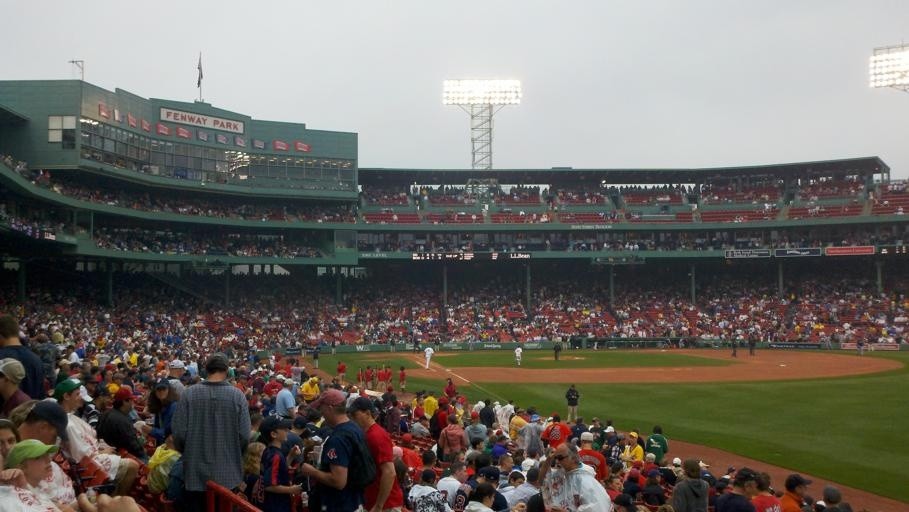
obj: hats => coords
[673,456,842,504]
[0,353,380,490]
[385,378,668,481]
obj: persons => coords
[2,192,356,258]
[422,345,435,371]
[307,260,909,349]
[312,350,319,369]
[514,345,523,366]
[729,329,738,358]
[1,266,306,511]
[301,361,854,511]
[553,342,562,361]
[355,174,909,254]
[1,155,306,221]
[747,329,756,357]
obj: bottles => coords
[85,486,97,504]
[138,434,146,444]
[129,405,138,419]
[146,418,154,426]
[301,492,309,507]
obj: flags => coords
[196,54,204,87]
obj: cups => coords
[308,452,316,466]
